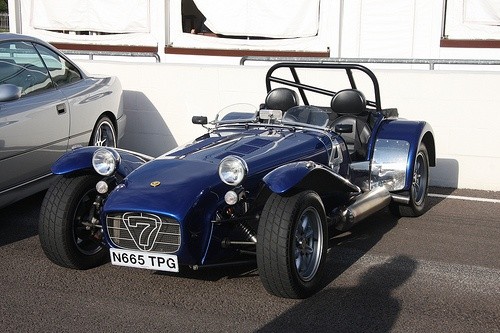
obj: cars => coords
[0,32,128,213]
[37,61,437,301]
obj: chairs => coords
[262,88,299,124]
[328,89,366,160]
[0,59,19,85]
